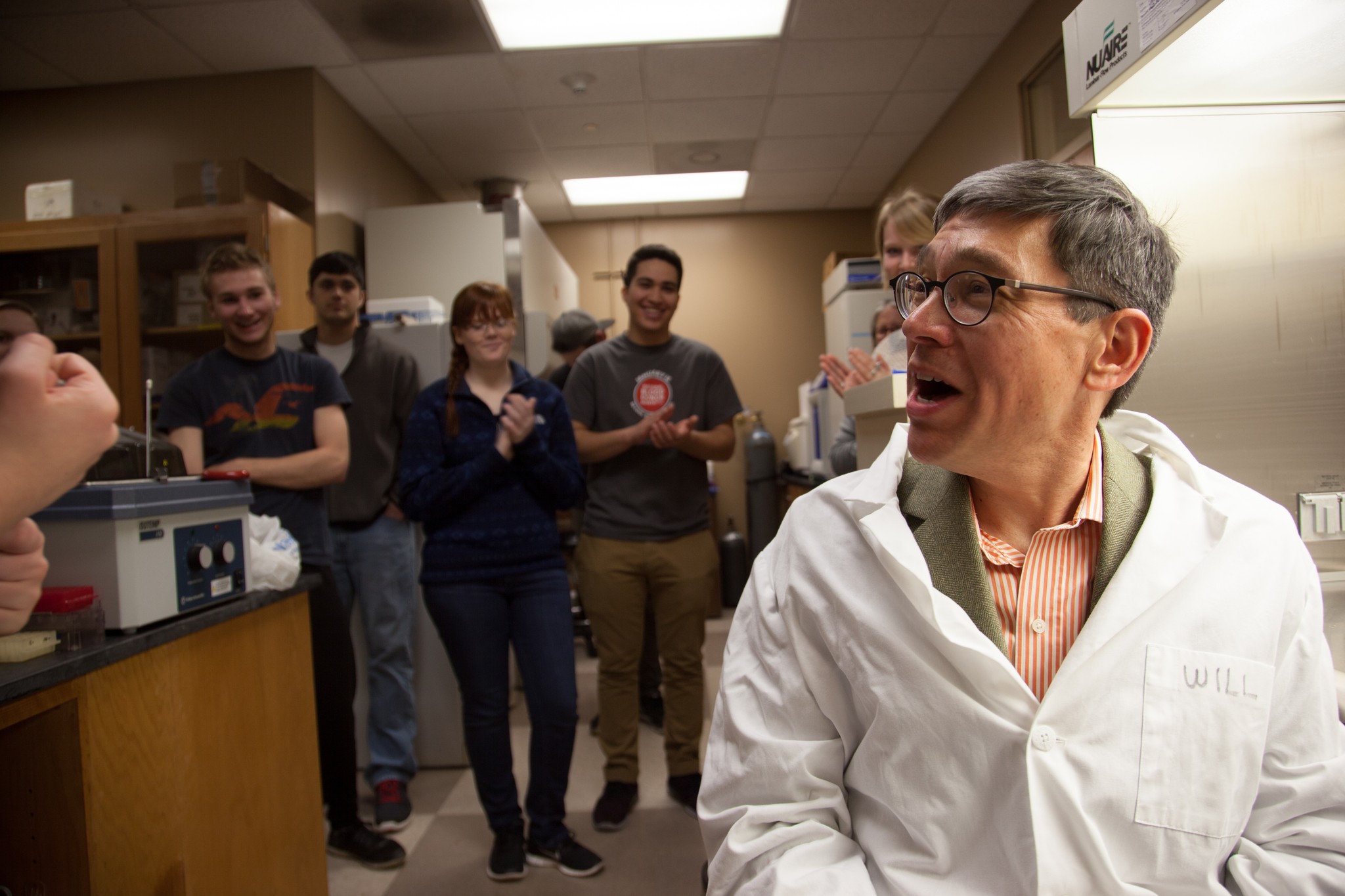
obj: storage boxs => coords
[778,250,911,479]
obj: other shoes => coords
[590,714,599,732]
[637,699,662,731]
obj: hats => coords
[552,311,617,351]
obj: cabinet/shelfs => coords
[114,200,316,431]
[361,187,579,771]
[0,219,121,420]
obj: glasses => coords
[888,269,1122,327]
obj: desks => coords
[0,574,335,896]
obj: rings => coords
[840,382,845,389]
[871,368,877,376]
[875,362,881,370]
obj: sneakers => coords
[666,771,701,811]
[325,820,405,868]
[371,777,412,824]
[486,833,525,880]
[592,779,639,833]
[525,829,604,878]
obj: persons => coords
[816,187,943,477]
[694,160,1345,896]
[296,247,427,835]
[151,241,406,872]
[0,298,120,635]
[535,242,743,831]
[392,280,605,881]
